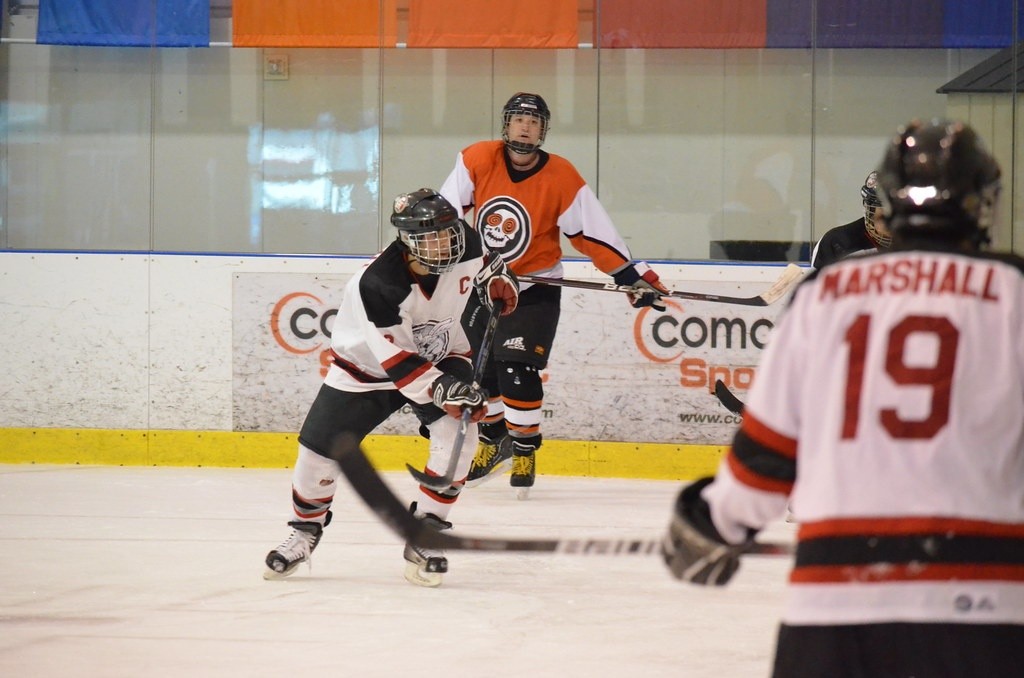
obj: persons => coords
[438,92,669,488]
[258,188,520,587]
[656,116,1024,678]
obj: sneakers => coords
[263,509,333,581]
[465,429,513,487]
[509,440,536,501]
[403,501,452,587]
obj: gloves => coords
[432,373,490,424]
[660,475,761,585]
[608,260,670,308]
[473,250,521,319]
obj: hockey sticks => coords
[328,430,797,556]
[405,299,505,491]
[714,378,749,416]
[515,262,804,307]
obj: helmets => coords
[861,170,893,248]
[502,91,551,155]
[391,188,466,275]
[875,118,1002,249]
